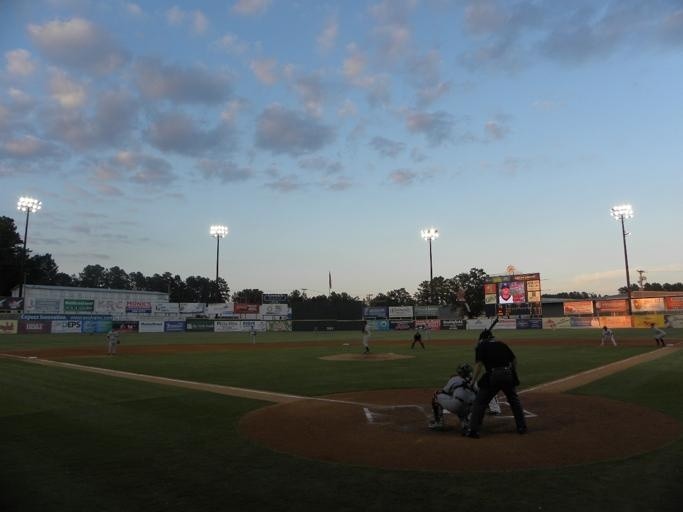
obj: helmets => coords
[455,363,473,378]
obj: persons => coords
[597,325,617,348]
[498,283,513,304]
[107,327,120,355]
[359,319,370,354]
[248,326,256,345]
[424,361,482,434]
[650,322,666,346]
[462,327,527,438]
[409,330,425,350]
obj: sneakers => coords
[426,416,529,436]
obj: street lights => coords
[17,197,41,297]
[610,205,634,315]
[421,228,439,305]
[210,225,228,294]
